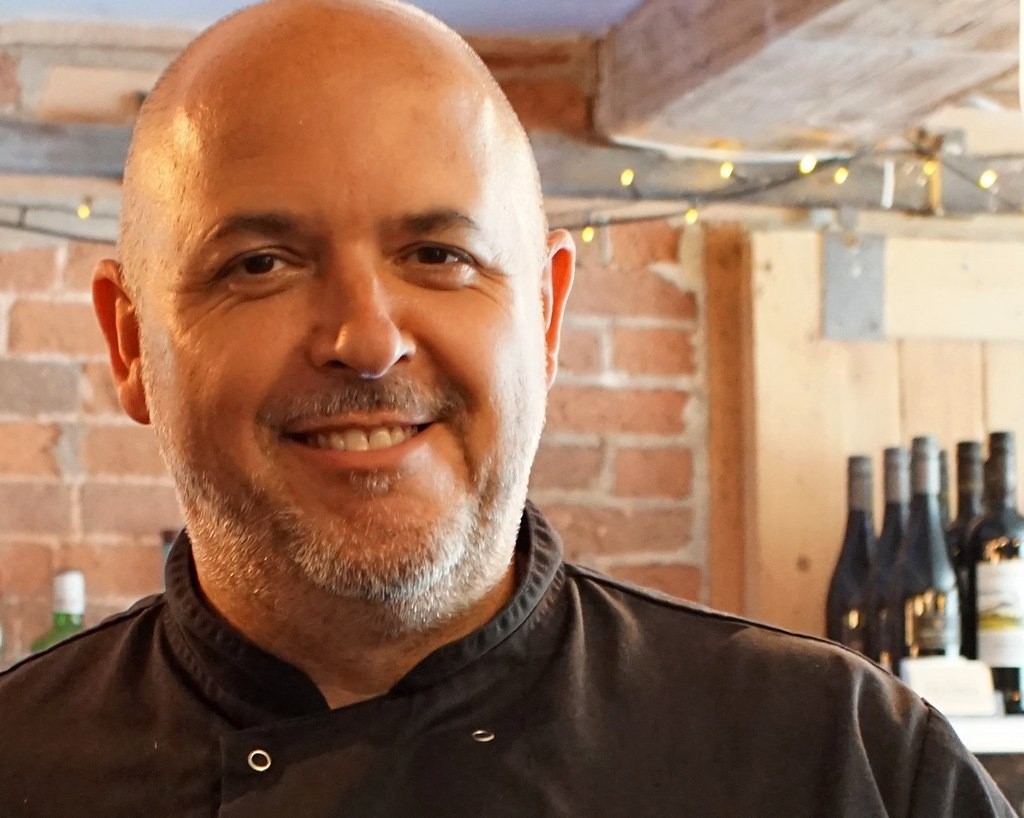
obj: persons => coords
[1,0,1016,818]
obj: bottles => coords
[31,569,92,655]
[826,430,1024,716]
